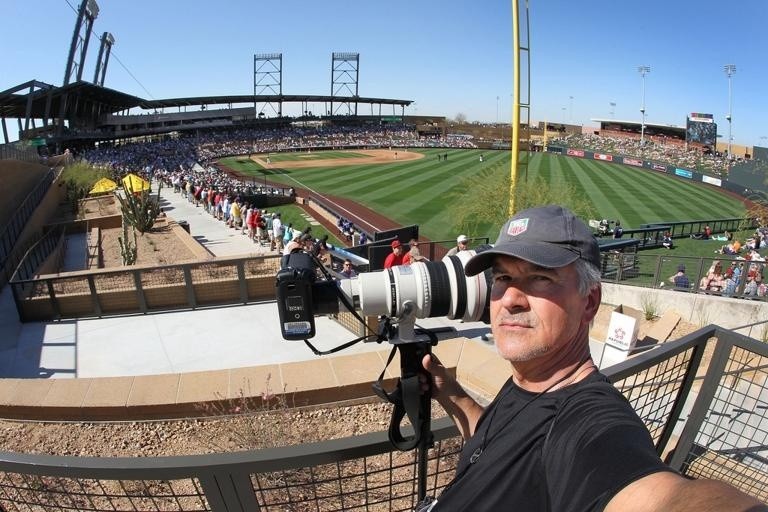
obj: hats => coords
[464,206,602,277]
[457,235,468,243]
[391,240,403,248]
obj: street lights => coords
[331,52,359,98]
[63,0,100,86]
[722,65,737,156]
[638,66,651,146]
[253,54,283,118]
[94,31,116,87]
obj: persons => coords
[384,240,402,270]
[41,120,476,199]
[338,216,367,244]
[411,206,768,512]
[480,155,482,164]
[445,235,468,257]
[476,237,493,253]
[339,259,355,278]
[538,133,748,181]
[172,173,328,256]
[690,226,710,240]
[282,241,316,278]
[712,231,733,241]
[668,264,686,285]
[403,238,431,265]
[661,230,672,250]
[691,218,767,300]
[395,151,397,160]
[612,220,623,264]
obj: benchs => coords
[336,223,350,240]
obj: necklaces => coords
[469,357,591,464]
